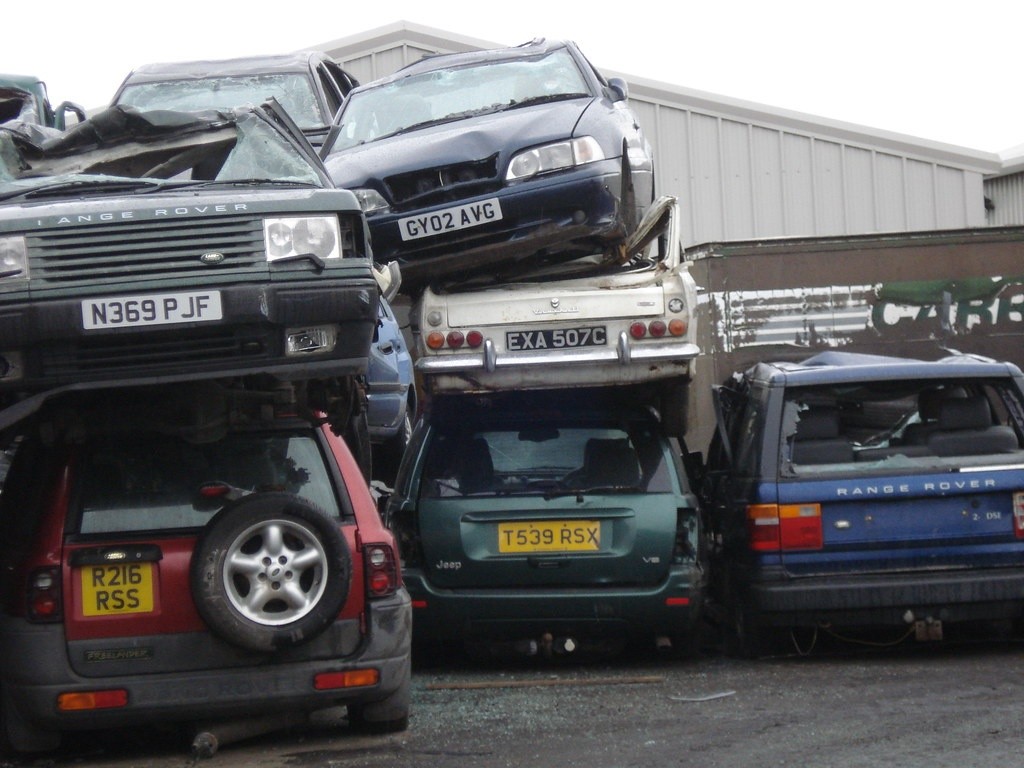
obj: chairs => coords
[925,397,1017,457]
[440,438,495,491]
[789,411,857,463]
[580,437,638,493]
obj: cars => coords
[409,197,701,435]
[318,37,655,288]
[0,50,416,462]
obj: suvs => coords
[385,397,709,668]
[703,351,1024,654]
[0,392,413,757]
